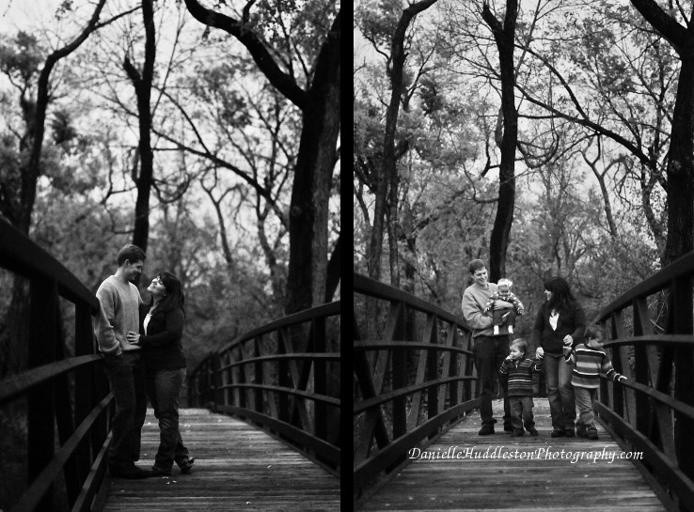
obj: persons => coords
[532,277,585,439]
[460,259,516,436]
[91,242,149,481]
[126,269,196,475]
[562,324,627,440]
[497,337,544,437]
[482,277,525,336]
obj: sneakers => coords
[503,419,600,441]
[115,450,194,480]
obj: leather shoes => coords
[477,424,496,436]
